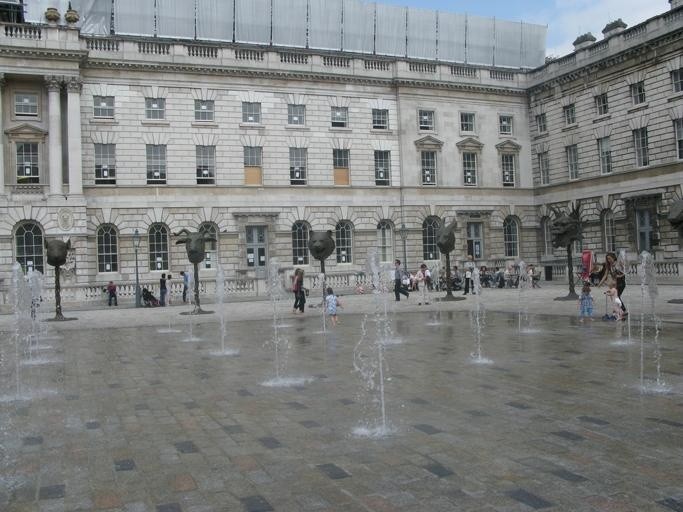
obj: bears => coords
[307,228,335,261]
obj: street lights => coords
[131,228,146,308]
[399,224,410,273]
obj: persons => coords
[107,281,117,306]
[293,268,300,313]
[605,279,622,320]
[165,275,172,306]
[598,252,628,316]
[297,269,306,313]
[589,263,608,287]
[323,287,345,326]
[577,284,596,323]
[179,270,189,302]
[142,289,159,304]
[159,274,167,306]
[354,259,542,305]
